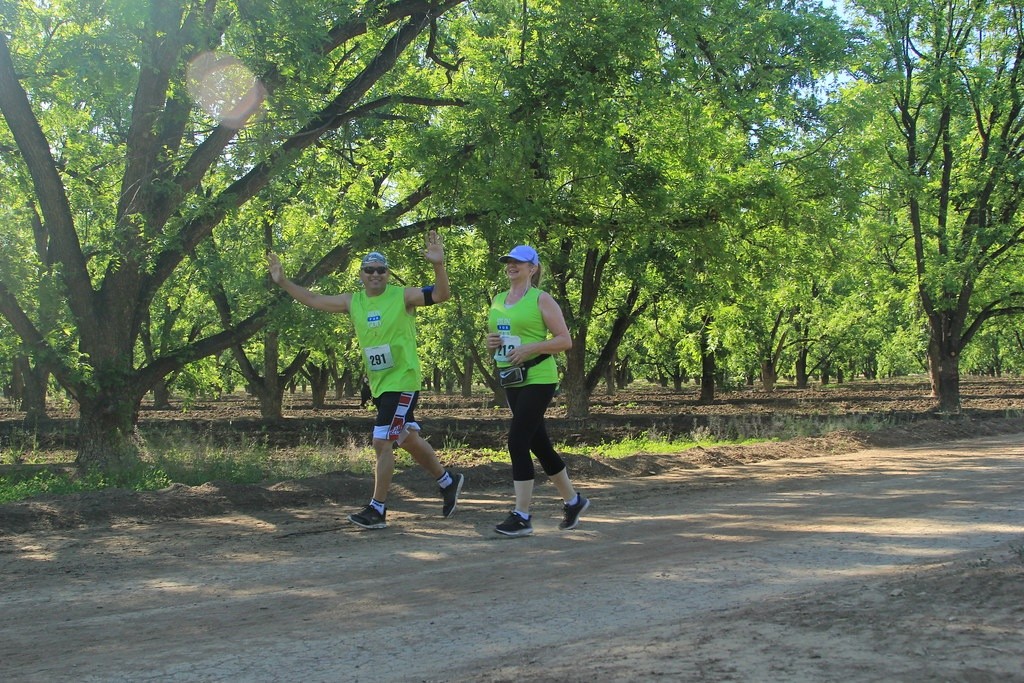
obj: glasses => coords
[361,266,388,274]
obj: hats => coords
[499,245,539,266]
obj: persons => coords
[265,231,464,528]
[487,246,589,536]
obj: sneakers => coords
[495,511,534,535]
[441,473,464,518]
[348,503,387,528]
[558,492,590,529]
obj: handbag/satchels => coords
[500,353,550,386]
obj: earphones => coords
[530,268,534,274]
